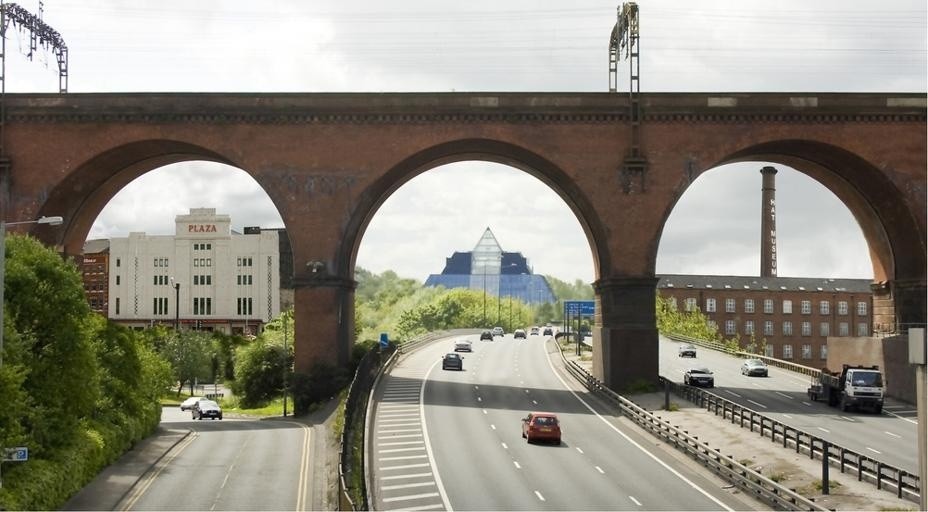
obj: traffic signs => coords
[564,300,595,316]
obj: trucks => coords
[820,364,888,414]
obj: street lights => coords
[170,277,181,328]
[1,216,65,372]
[244,227,287,235]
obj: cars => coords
[455,339,473,352]
[522,413,562,443]
[180,396,223,420]
[678,344,697,358]
[741,359,768,377]
[480,322,553,341]
[684,368,714,388]
[441,353,464,370]
